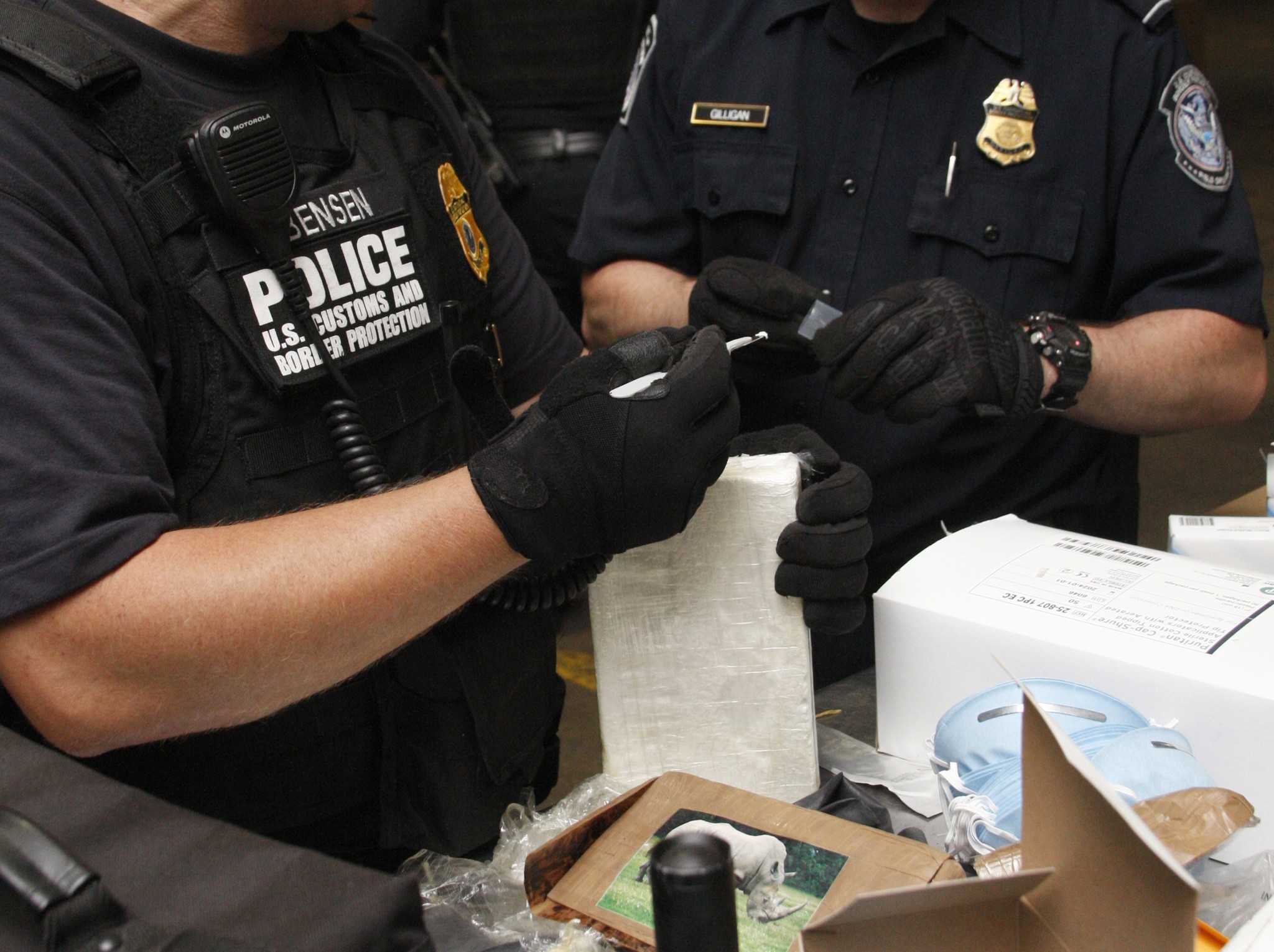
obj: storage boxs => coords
[522,514,1274,952]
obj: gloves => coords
[810,276,1043,426]
[466,323,739,568]
[689,257,832,385]
[724,424,873,633]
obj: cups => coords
[650,832,739,952]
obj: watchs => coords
[1019,310,1094,419]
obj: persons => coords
[0,0,877,877]
[572,0,1274,678]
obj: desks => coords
[423,481,1274,952]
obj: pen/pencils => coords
[607,331,769,399]
